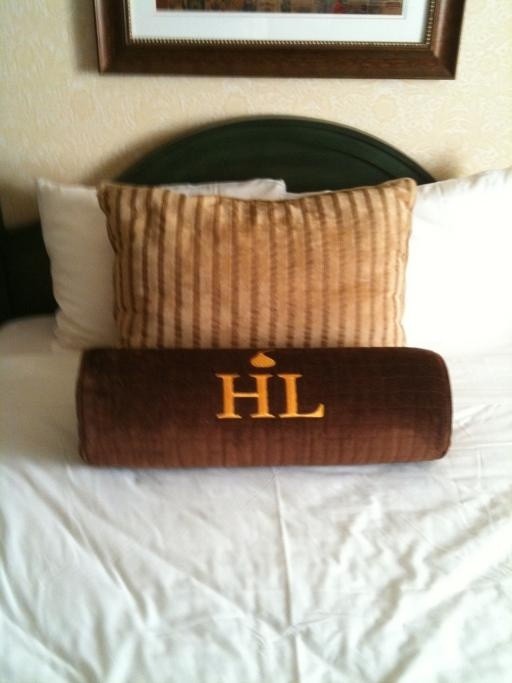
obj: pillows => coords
[96,177,414,350]
[32,169,290,350]
[77,346,452,465]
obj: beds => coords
[0,114,507,678]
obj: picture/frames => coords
[89,1,466,83]
